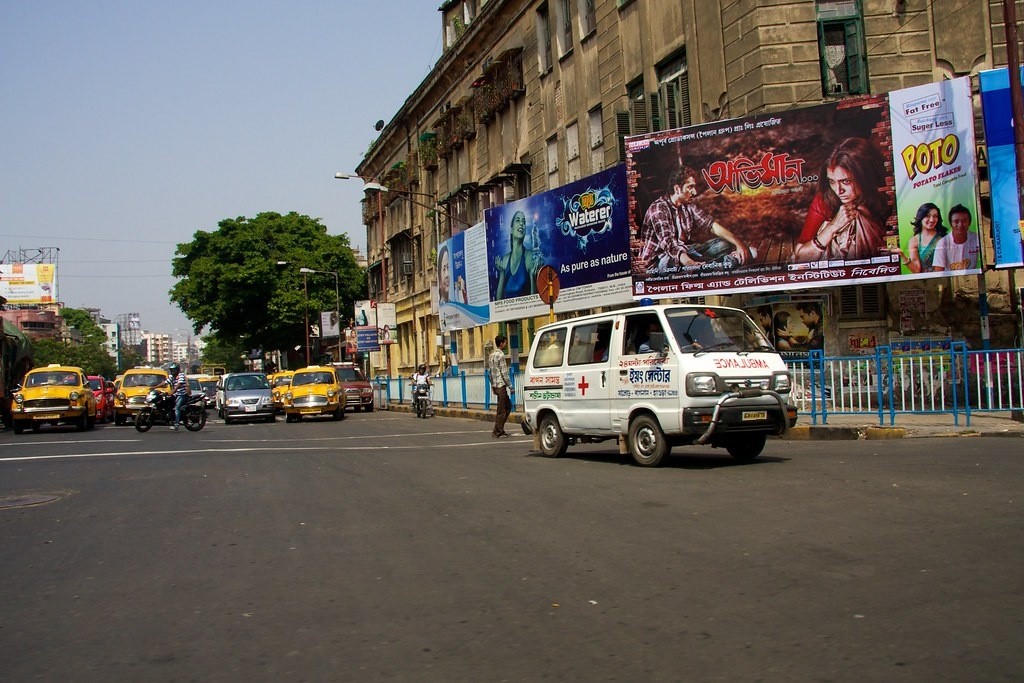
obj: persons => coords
[791,137,890,262]
[539,318,667,366]
[438,246,451,302]
[384,325,391,340]
[347,330,357,348]
[489,335,515,439]
[155,363,187,431]
[641,165,757,279]
[773,302,823,368]
[743,305,774,349]
[413,364,435,417]
[882,203,980,273]
[495,210,539,300]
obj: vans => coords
[524,304,798,465]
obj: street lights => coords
[336,172,391,377]
[363,182,445,373]
[301,268,342,363]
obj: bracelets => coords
[811,237,826,252]
[903,258,911,265]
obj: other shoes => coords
[175,425,179,431]
[492,434,508,438]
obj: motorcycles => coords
[409,384,434,419]
[135,389,207,433]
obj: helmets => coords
[419,364,427,370]
[169,362,180,376]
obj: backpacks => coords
[184,376,192,395]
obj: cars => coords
[326,362,374,414]
[113,369,171,426]
[187,374,220,408]
[283,366,346,422]
[86,375,123,423]
[11,364,96,434]
[215,373,274,425]
[267,371,295,415]
